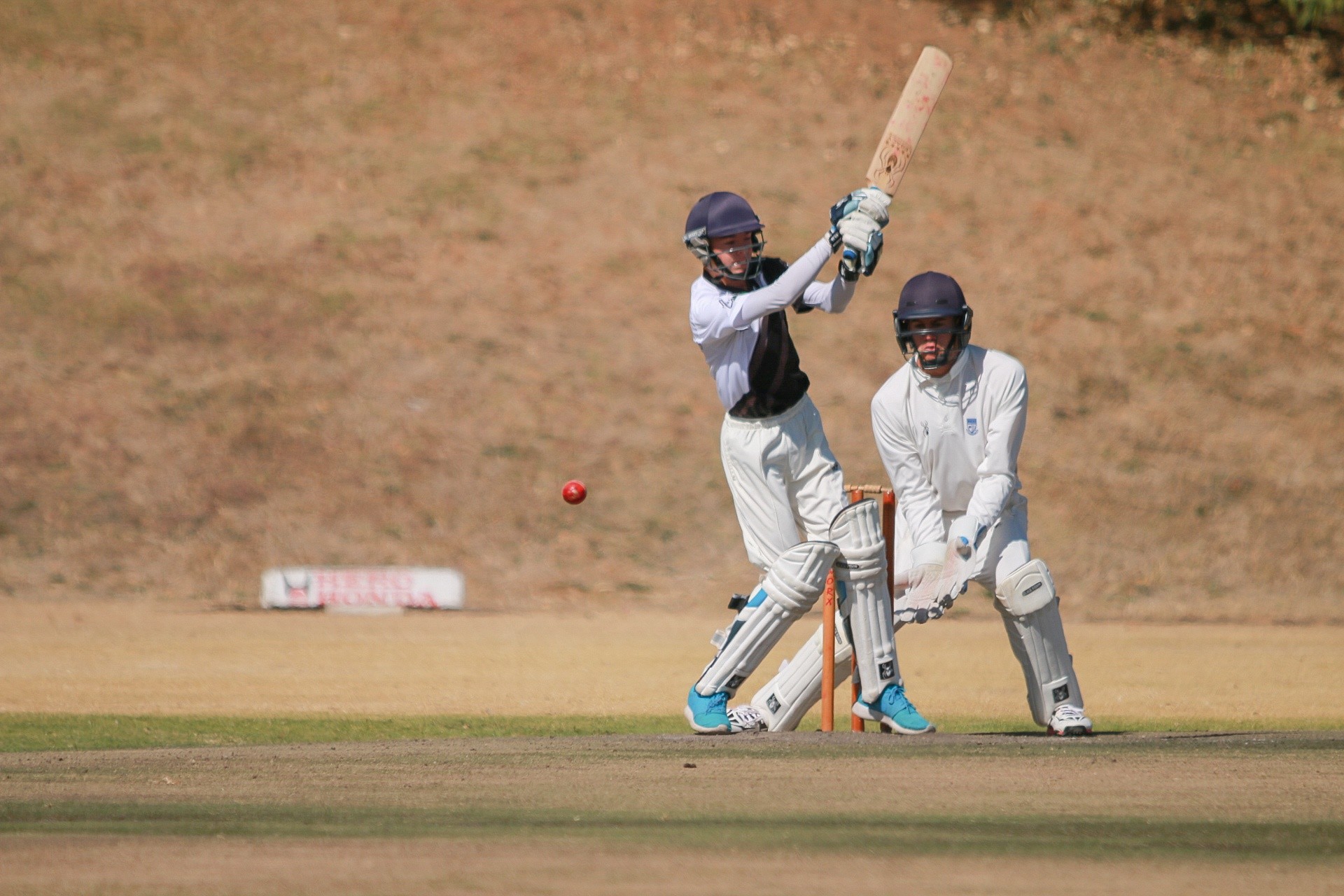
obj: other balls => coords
[561,479,587,505]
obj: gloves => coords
[825,188,891,253]
[899,543,968,623]
[839,213,883,281]
[930,515,988,608]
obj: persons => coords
[683,185,938,737]
[726,272,1091,737]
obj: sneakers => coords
[685,683,732,734]
[1047,705,1093,737]
[852,683,936,735]
[726,705,768,733]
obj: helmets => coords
[681,191,765,249]
[897,271,966,319]
